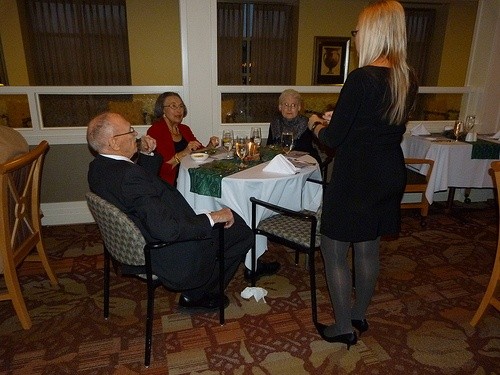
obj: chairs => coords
[0,138,57,331]
[84,183,226,369]
[471,160,500,326]
[401,158,434,226]
[249,177,325,321]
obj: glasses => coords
[282,103,298,109]
[351,30,359,36]
[162,104,185,109]
[108,127,134,146]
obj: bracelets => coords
[311,121,321,131]
[175,153,181,164]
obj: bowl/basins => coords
[190,153,209,161]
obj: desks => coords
[176,146,322,272]
[400,132,500,207]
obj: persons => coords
[147,92,219,187]
[308,0,418,350]
[266,89,314,154]
[86,113,281,312]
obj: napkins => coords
[263,154,300,174]
[410,122,431,136]
[491,130,500,141]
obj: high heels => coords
[313,321,358,350]
[352,319,369,336]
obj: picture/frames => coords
[312,36,351,85]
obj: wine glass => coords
[280,132,294,158]
[221,127,263,167]
[452,122,463,143]
[465,115,476,134]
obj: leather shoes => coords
[244,257,281,283]
[178,293,229,312]
